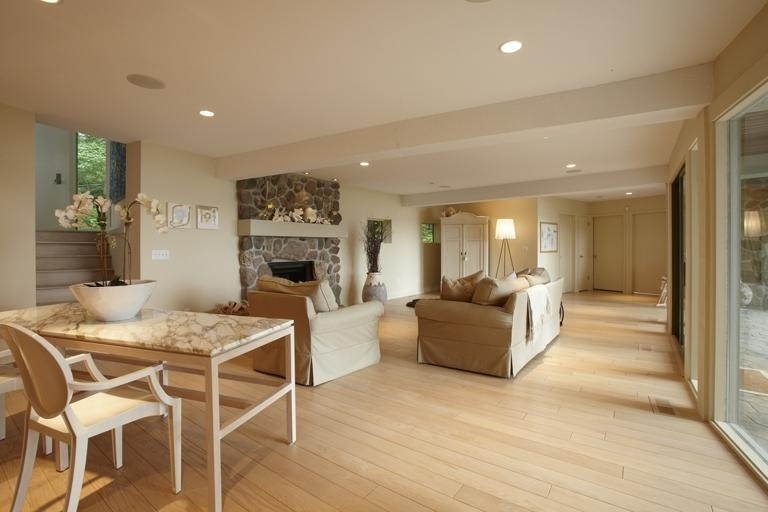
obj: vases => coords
[69,280,158,321]
[362,272,387,305]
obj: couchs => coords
[414,277,564,378]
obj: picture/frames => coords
[540,222,558,252]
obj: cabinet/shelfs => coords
[440,212,489,291]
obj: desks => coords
[1,302,297,512]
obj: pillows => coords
[441,268,550,306]
[257,276,338,312]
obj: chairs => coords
[248,291,384,387]
[0,322,182,512]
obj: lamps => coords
[495,219,514,278]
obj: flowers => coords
[55,191,168,287]
